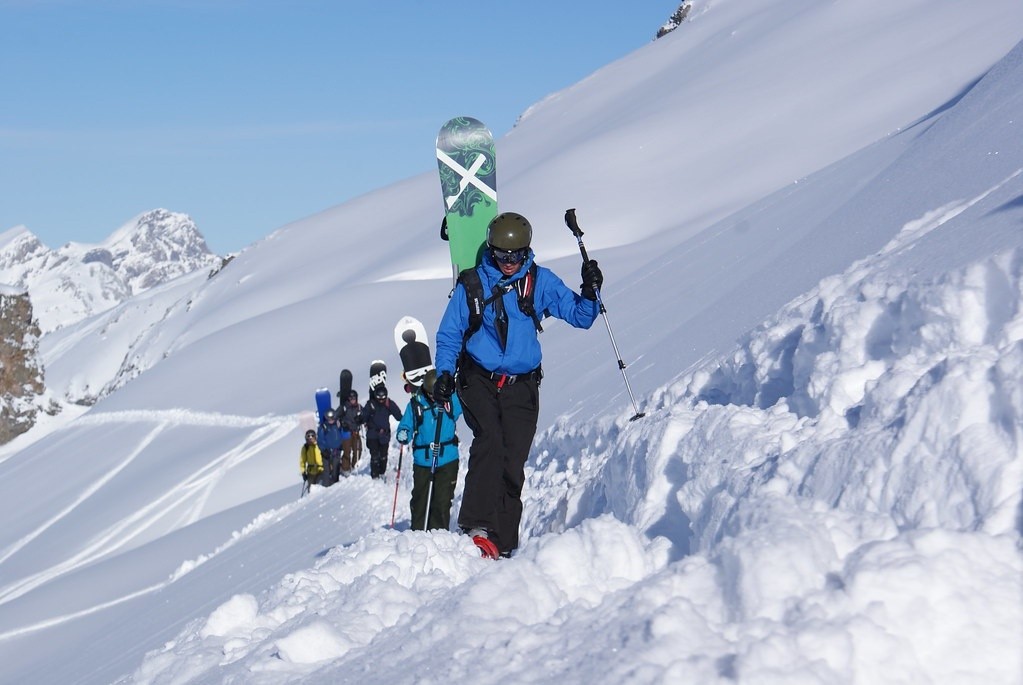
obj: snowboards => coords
[339,368,353,406]
[315,387,332,426]
[393,314,435,399]
[368,359,388,401]
[434,115,502,294]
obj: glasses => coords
[307,435,315,438]
[489,246,528,264]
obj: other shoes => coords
[463,526,489,540]
[499,549,512,559]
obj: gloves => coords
[580,260,603,301]
[303,474,308,481]
[355,415,361,423]
[322,449,330,459]
[433,374,455,404]
[398,430,408,442]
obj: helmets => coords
[424,369,438,396]
[489,212,532,251]
[374,387,387,401]
[324,408,336,417]
[306,430,315,436]
[346,390,358,397]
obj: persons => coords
[335,390,367,476]
[317,408,342,487]
[355,387,404,483]
[396,369,463,532]
[300,429,323,493]
[434,212,603,559]
